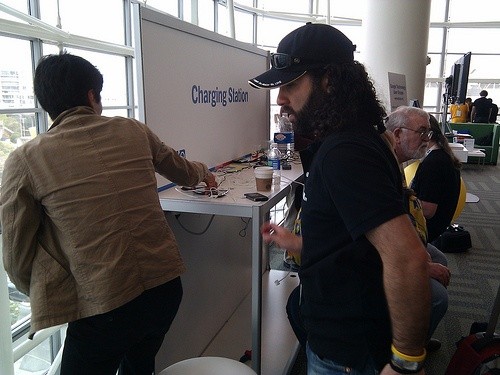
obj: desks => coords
[157,156,305,375]
[468,148,486,170]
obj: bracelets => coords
[388,360,423,375]
[391,343,427,362]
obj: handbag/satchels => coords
[438,224,472,253]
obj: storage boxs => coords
[282,164,292,170]
[277,144,287,155]
[274,132,295,144]
[452,146,468,163]
[448,142,464,150]
[272,160,280,170]
[453,136,471,144]
[464,139,475,152]
[290,143,294,156]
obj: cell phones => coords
[244,193,269,201]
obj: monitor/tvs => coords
[446,52,471,104]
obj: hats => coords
[249,23,356,90]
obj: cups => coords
[254,167,273,192]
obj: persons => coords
[382,105,451,356]
[412,114,466,246]
[0,52,218,375]
[450,90,498,123]
[248,21,432,375]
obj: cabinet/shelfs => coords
[438,122,500,167]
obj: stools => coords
[158,357,257,375]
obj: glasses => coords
[400,127,433,139]
[270,53,319,71]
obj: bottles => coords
[278,113,292,154]
[267,143,282,190]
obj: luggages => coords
[443,286,500,375]
